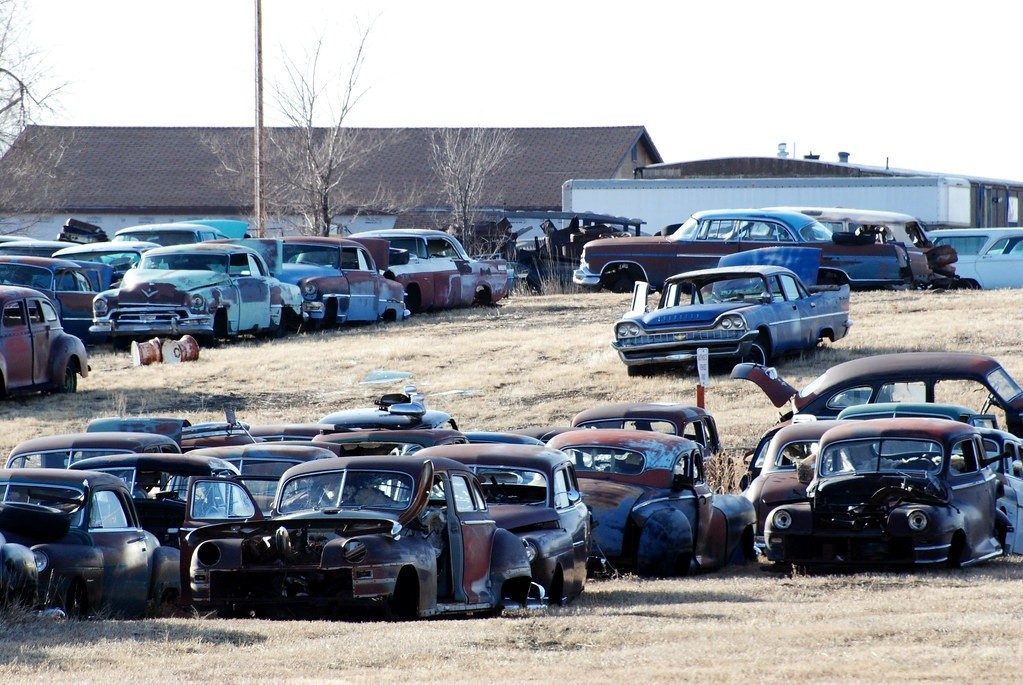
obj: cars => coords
[0,217,1023,621]
[570,207,913,294]
[1,283,92,405]
[924,227,1023,290]
[609,246,856,377]
[725,205,961,289]
